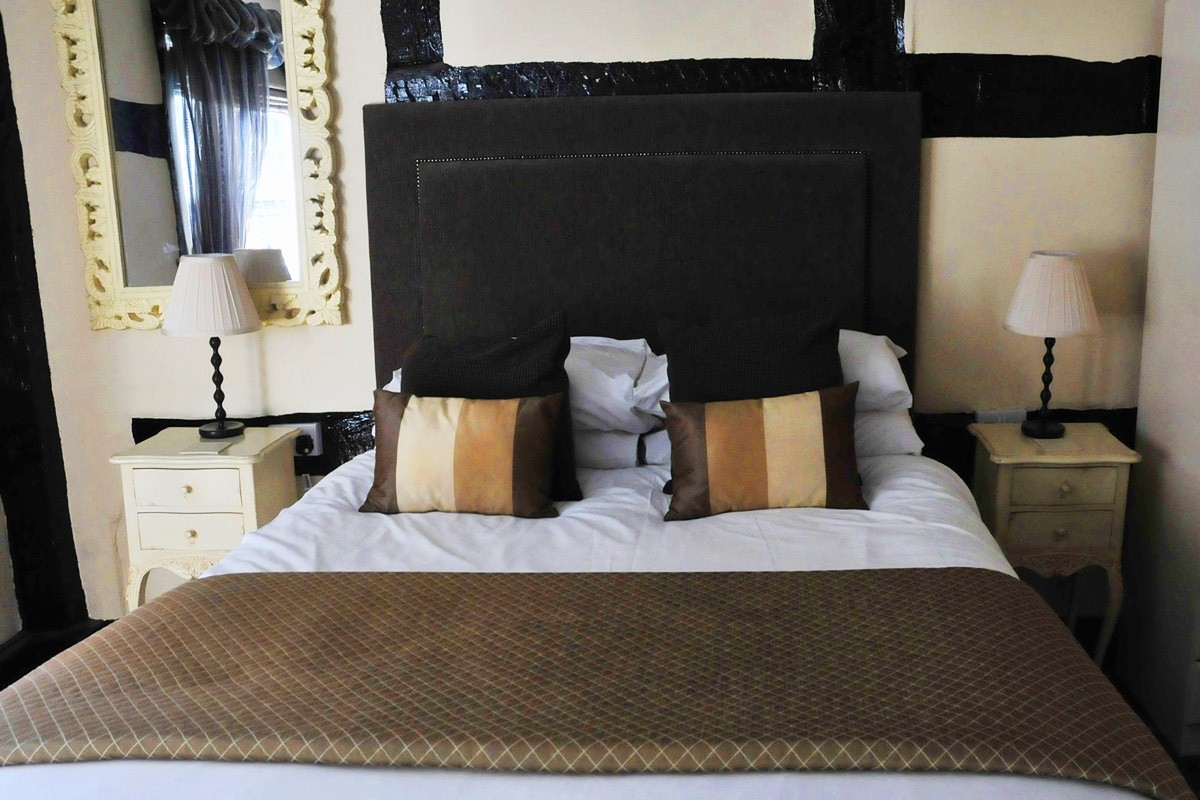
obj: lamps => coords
[159,254,264,440]
[1002,250,1103,439]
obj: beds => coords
[0,90,1189,800]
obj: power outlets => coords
[267,423,323,457]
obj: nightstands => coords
[967,422,1143,671]
[106,424,303,614]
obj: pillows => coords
[400,324,584,502]
[631,333,915,411]
[357,389,563,519]
[381,336,660,433]
[659,381,868,520]
[662,304,867,497]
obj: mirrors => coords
[50,0,347,330]
